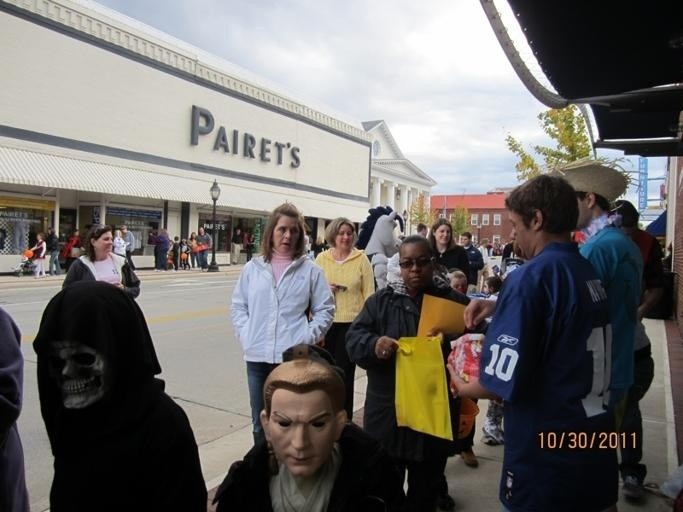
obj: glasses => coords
[399,254,430,269]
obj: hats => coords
[549,155,648,213]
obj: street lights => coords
[402,209,408,234]
[205,179,221,272]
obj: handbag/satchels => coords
[121,262,140,287]
[394,336,454,442]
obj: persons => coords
[1,279,210,510]
[445,175,619,512]
[555,154,645,455]
[62,224,141,299]
[218,359,394,512]
[13,223,212,273]
[229,203,664,509]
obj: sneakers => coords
[461,450,478,467]
[481,434,498,445]
[623,475,641,498]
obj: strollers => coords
[11,257,36,278]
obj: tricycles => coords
[166,253,190,271]
